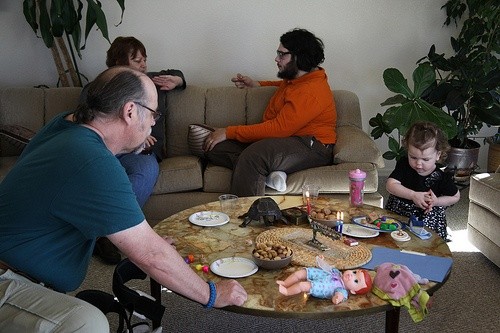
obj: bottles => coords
[349,169,367,207]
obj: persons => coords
[275,268,371,305]
[385,121,461,242]
[0,65,248,333]
[93,37,186,265]
[202,28,336,197]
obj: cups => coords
[218,194,238,215]
[301,184,319,204]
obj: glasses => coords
[276,50,292,57]
[133,101,162,121]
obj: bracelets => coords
[202,279,216,309]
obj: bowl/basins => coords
[309,216,337,227]
[251,246,293,269]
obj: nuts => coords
[254,241,292,261]
[309,206,342,221]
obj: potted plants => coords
[369,0,500,182]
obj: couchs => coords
[0,85,387,228]
[466,172,500,268]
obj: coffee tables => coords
[151,196,453,333]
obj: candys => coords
[183,254,209,273]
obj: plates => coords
[188,211,230,227]
[352,215,407,233]
[338,223,379,238]
[209,257,259,278]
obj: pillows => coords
[187,123,217,163]
[0,126,37,157]
